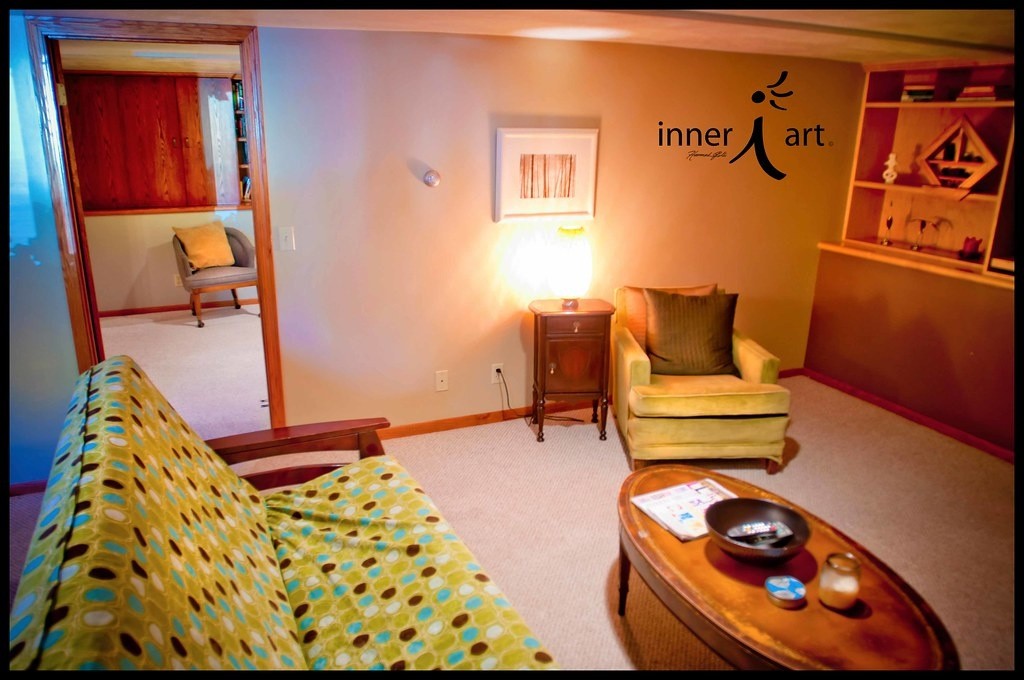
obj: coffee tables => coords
[616,467,962,670]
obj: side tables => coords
[527,299,614,442]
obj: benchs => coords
[9,353,556,671]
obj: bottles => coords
[818,552,860,611]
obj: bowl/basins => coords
[704,498,811,564]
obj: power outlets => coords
[492,363,504,384]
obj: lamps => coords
[551,225,592,311]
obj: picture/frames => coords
[494,127,600,224]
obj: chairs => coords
[173,227,262,328]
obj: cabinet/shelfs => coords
[808,55,1016,464]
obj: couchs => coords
[615,283,791,476]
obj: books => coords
[900,72,949,101]
[240,176,251,198]
[956,86,998,101]
[630,477,741,542]
[241,142,248,164]
[236,116,246,138]
[232,82,244,111]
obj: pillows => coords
[172,220,235,269]
[642,287,743,378]
